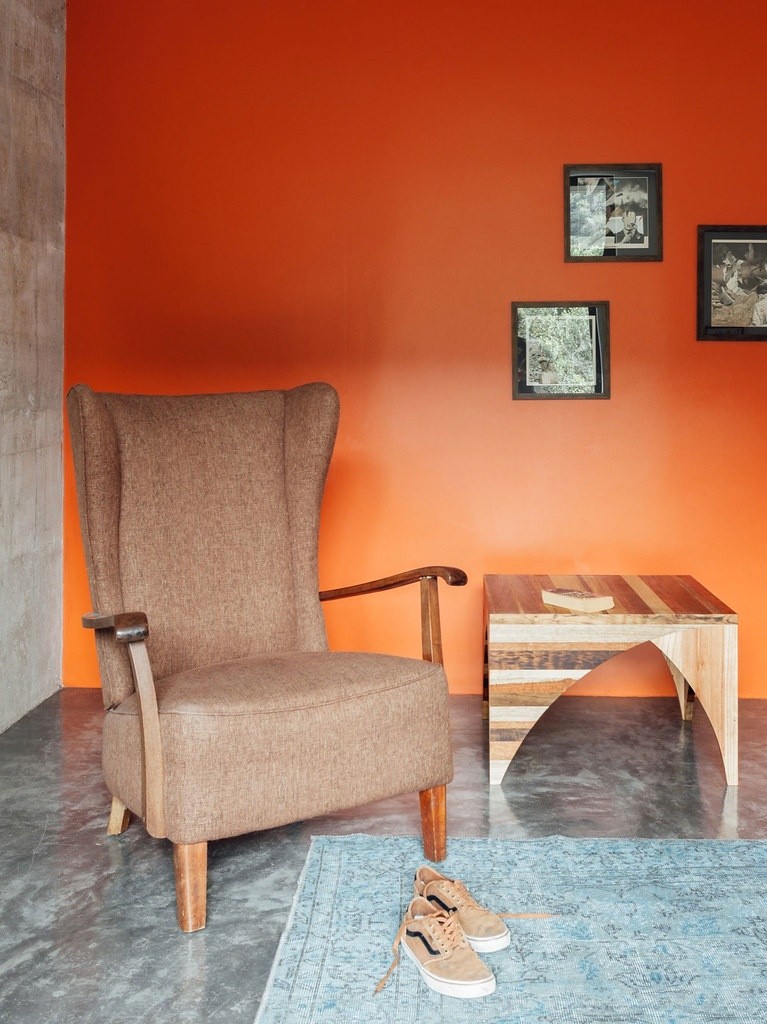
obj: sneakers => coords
[372,896,496,998]
[413,865,511,953]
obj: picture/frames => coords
[696,224,767,341]
[510,300,611,400]
[564,163,663,262]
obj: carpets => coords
[252,833,767,1024]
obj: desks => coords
[484,574,739,787]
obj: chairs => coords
[66,383,468,933]
